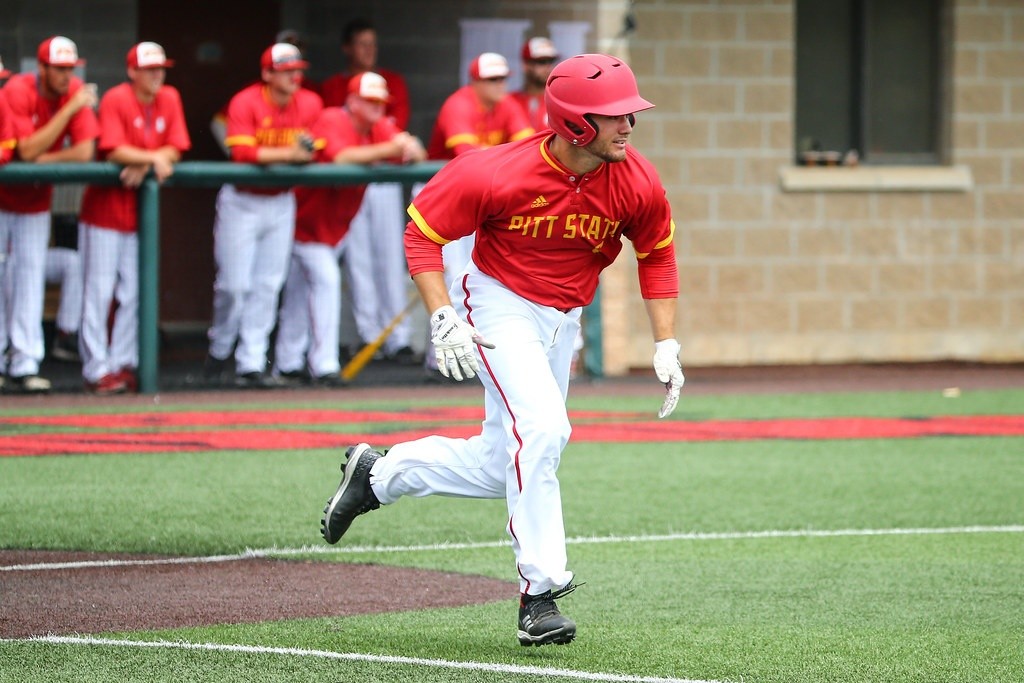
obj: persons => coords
[413,37,564,379]
[0,37,190,395]
[320,53,683,648]
[204,25,428,389]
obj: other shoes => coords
[95,368,137,394]
[17,375,51,393]
[0,373,20,393]
[204,345,426,389]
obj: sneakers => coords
[517,574,587,646]
[320,442,389,544]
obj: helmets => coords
[544,54,656,147]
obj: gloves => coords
[652,339,685,418]
[430,304,496,381]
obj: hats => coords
[260,43,309,71]
[521,37,561,62]
[346,71,389,103]
[125,42,175,69]
[36,36,87,66]
[468,52,513,81]
